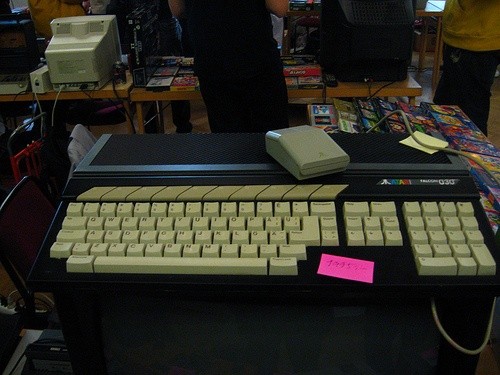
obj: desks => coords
[0,70,135,135]
[283,8,446,96]
[130,55,422,135]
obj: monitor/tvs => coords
[44,15,122,91]
[319,0,414,81]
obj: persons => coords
[28,0,192,136]
[168,0,289,134]
[432,0,500,138]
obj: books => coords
[149,55,200,93]
[307,98,486,137]
[446,137,500,235]
[281,53,321,89]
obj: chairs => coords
[0,175,59,312]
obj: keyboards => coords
[24,176,500,288]
